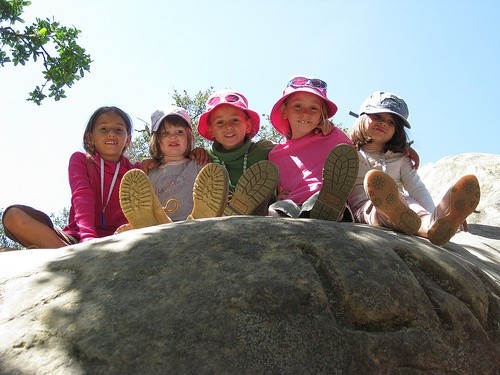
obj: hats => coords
[149,107,193,132]
[197,92,260,141]
[349,91,411,129]
[269,81,337,135]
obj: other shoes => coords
[186,163,228,220]
[222,160,279,217]
[118,168,178,229]
[310,144,360,222]
[426,175,481,246]
[363,169,421,235]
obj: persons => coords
[347,90,480,246]
[198,90,278,216]
[269,77,360,222]
[119,107,230,229]
[2,107,212,249]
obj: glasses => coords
[287,77,327,93]
